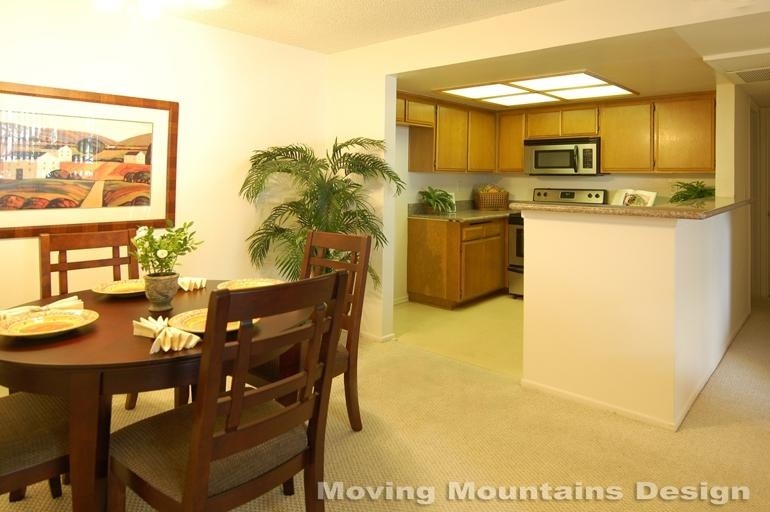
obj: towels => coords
[1,295,83,321]
[133,315,203,351]
[177,278,208,292]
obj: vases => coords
[143,274,179,313]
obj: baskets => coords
[474,191,509,210]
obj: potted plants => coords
[419,187,455,215]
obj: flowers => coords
[127,220,203,277]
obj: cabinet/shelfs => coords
[496,111,533,175]
[524,105,598,172]
[409,104,501,174]
[598,89,719,173]
[395,93,436,130]
[406,208,509,310]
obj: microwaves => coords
[524,136,601,176]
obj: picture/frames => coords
[2,81,180,240]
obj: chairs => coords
[109,270,348,510]
[0,388,83,492]
[39,228,141,410]
[297,229,370,432]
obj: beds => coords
[0,280,317,512]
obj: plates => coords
[216,278,284,289]
[0,308,100,341]
[91,279,145,297]
[167,308,261,333]
